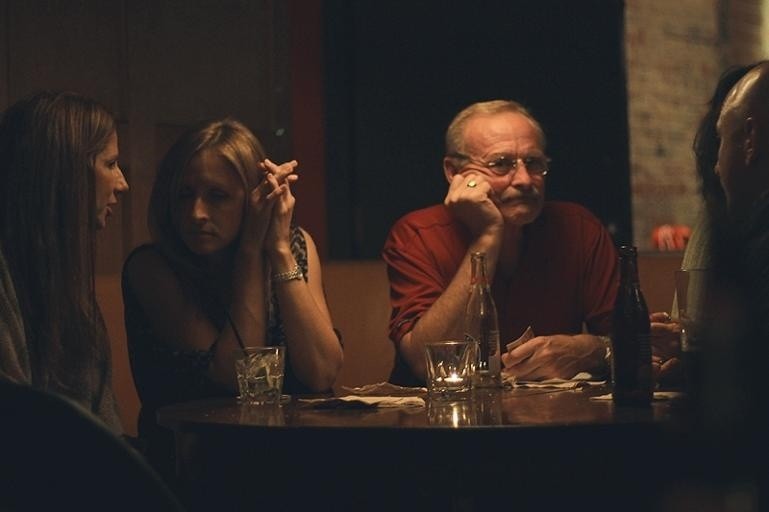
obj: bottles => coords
[464,248,502,390]
[606,246,654,406]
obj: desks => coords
[156,382,690,512]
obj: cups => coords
[235,346,287,406]
[425,339,478,403]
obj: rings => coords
[467,178,478,187]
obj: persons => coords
[380,100,626,383]
[1,91,130,441]
[653,58,769,403]
[121,118,345,439]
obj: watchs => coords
[269,266,305,283]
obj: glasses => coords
[439,152,552,176]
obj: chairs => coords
[2,377,175,512]
